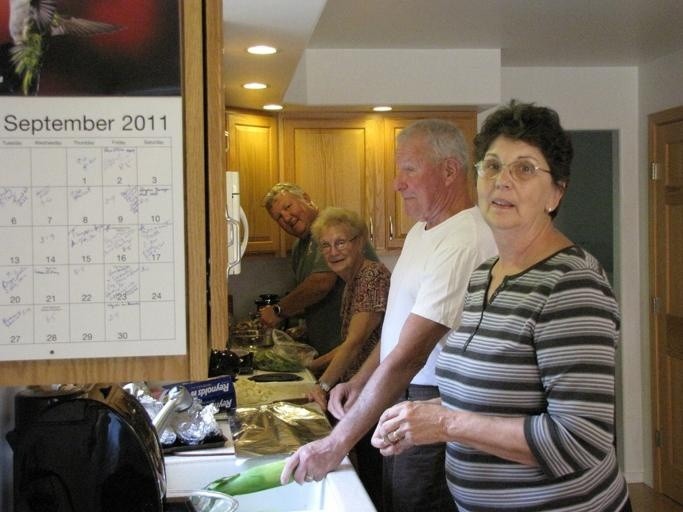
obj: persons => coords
[281,119,498,510]
[305,208,391,510]
[371,101,633,511]
[258,182,380,380]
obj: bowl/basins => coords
[229,349,249,359]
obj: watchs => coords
[273,302,282,318]
[317,380,331,391]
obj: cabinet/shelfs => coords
[225,115,280,254]
[284,117,475,256]
[1,1,228,388]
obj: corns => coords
[203,459,295,496]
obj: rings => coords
[393,432,401,442]
[306,476,314,481]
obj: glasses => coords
[318,234,361,253]
[473,157,552,182]
